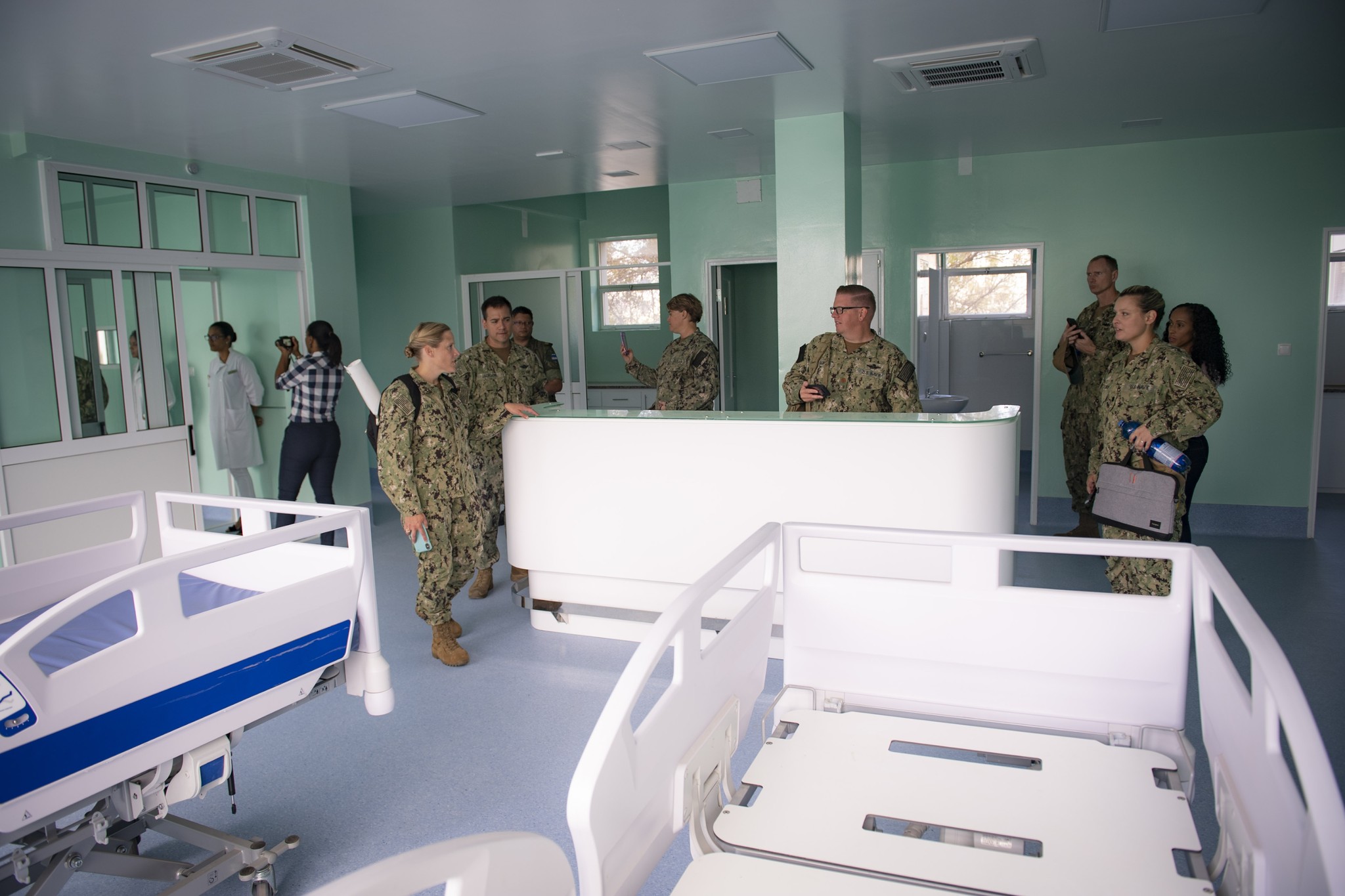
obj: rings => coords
[406,528,410,533]
[1140,440,1145,444]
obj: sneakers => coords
[510,565,528,582]
[468,568,493,599]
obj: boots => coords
[448,617,462,638]
[431,622,470,667]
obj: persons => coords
[620,293,720,411]
[447,296,550,599]
[377,322,538,666]
[1086,285,1224,596]
[1161,302,1233,544]
[509,306,563,403]
[782,284,924,413]
[205,321,265,536]
[275,320,345,546]
[74,355,109,424]
[1052,255,1129,538]
[129,330,176,431]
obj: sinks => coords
[918,394,969,414]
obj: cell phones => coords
[409,522,432,553]
[1084,485,1097,504]
[1066,318,1084,338]
[620,332,630,355]
[805,383,831,396]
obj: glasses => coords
[513,321,532,326]
[204,334,224,342]
[830,306,870,315]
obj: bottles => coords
[1118,419,1191,474]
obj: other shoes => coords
[226,525,243,536]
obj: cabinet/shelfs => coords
[587,388,657,410]
[1316,392,1345,493]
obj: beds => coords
[0,491,396,896]
[301,521,1345,896]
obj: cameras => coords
[275,336,294,348]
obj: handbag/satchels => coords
[783,332,837,419]
[1090,448,1181,541]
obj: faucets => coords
[925,386,939,398]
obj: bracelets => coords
[295,355,303,359]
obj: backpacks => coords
[366,373,457,458]
